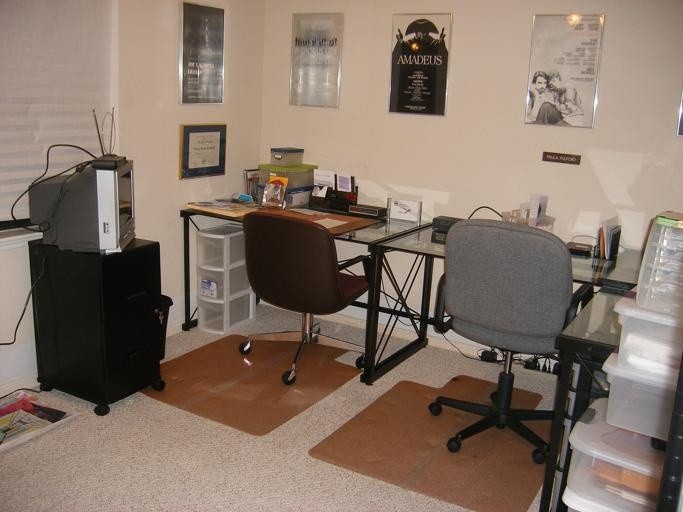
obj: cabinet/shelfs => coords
[28,237,165,415]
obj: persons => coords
[545,71,585,117]
[528,67,577,124]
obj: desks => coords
[178,199,429,370]
[363,226,645,435]
[537,289,683,512]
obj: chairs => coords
[427,219,595,464]
[237,212,382,384]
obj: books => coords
[591,258,613,285]
[501,192,550,228]
[625,354,679,379]
[622,333,683,369]
[592,217,623,259]
[587,462,661,510]
[654,210,683,230]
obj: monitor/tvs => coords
[28,160,135,255]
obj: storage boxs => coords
[557,296,683,512]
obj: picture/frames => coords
[180,1,225,105]
[287,12,345,110]
[522,11,606,130]
[180,123,226,182]
[388,10,454,117]
